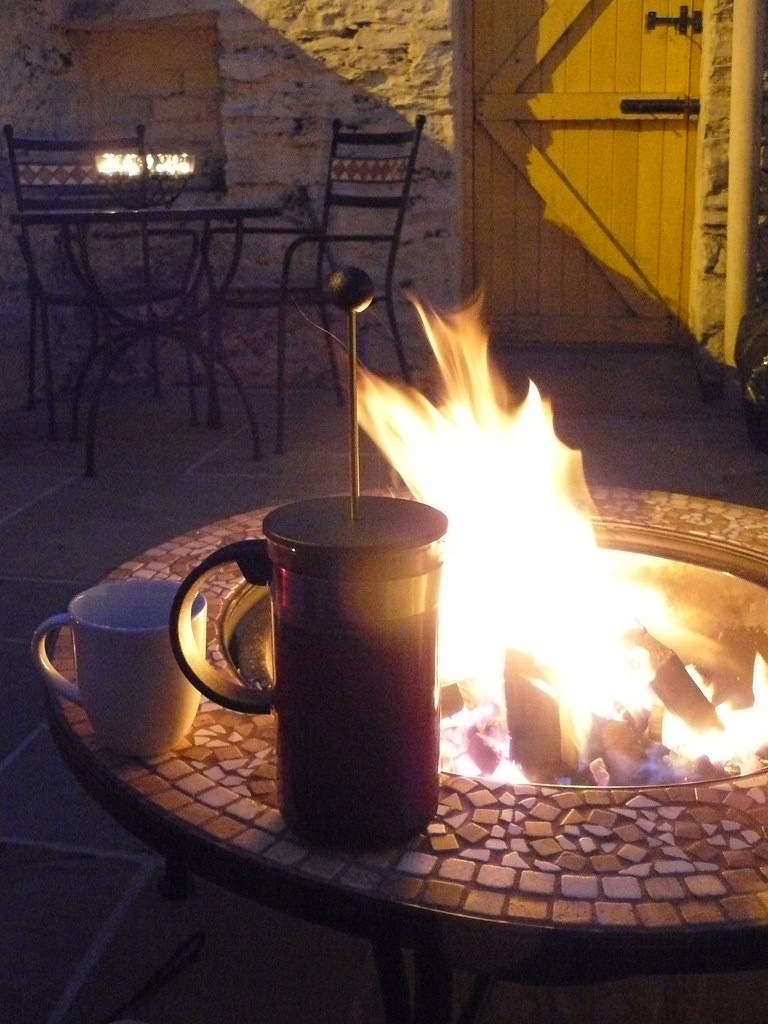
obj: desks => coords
[10,199,284,479]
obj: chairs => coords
[204,115,427,456]
[2,123,199,444]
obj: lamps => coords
[92,149,197,210]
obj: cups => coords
[31,581,206,758]
[171,498,449,848]
